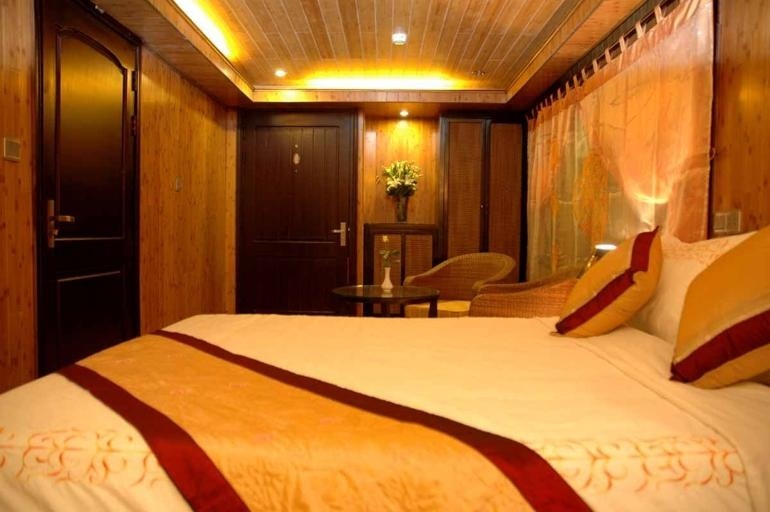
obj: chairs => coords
[403,251,519,317]
[470,267,582,318]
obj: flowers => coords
[377,235,400,269]
[375,158,423,197]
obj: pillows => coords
[624,229,754,346]
[669,224,769,391]
[547,220,663,340]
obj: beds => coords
[2,314,769,512]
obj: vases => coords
[393,192,410,223]
[381,268,394,293]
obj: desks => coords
[334,285,440,318]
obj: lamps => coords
[391,23,408,45]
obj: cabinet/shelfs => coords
[362,222,440,319]
[435,112,528,286]
